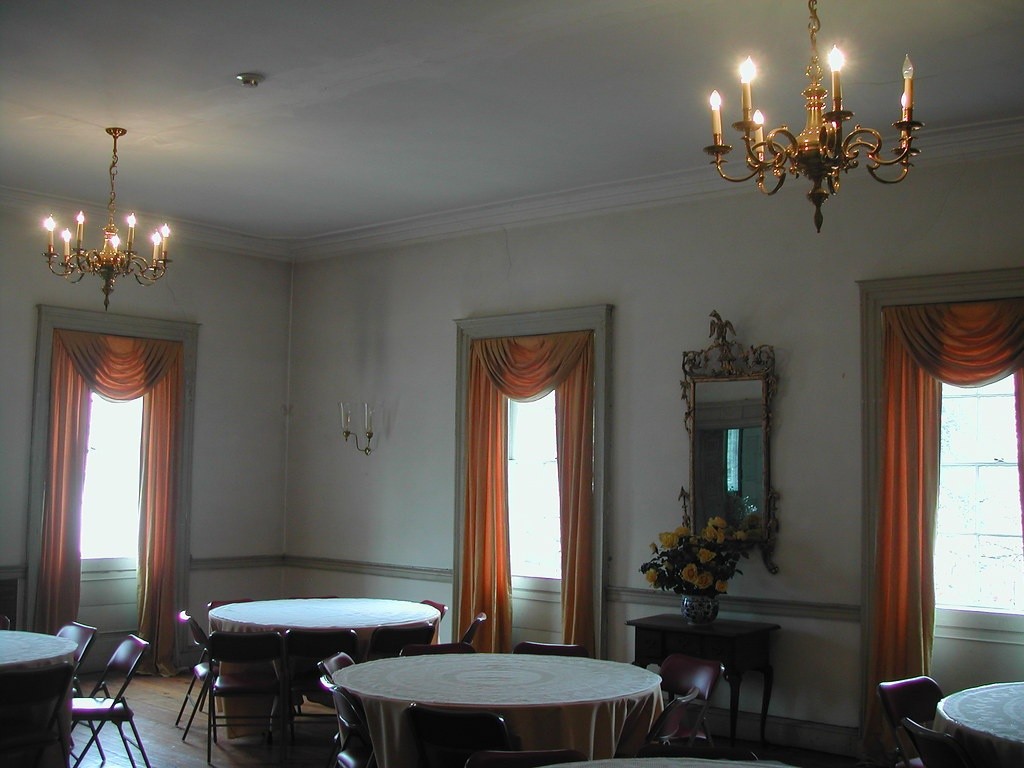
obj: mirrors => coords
[679,308,782,576]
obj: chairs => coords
[878,676,945,768]
[56,620,105,760]
[174,594,725,768]
[71,633,151,768]
[900,716,979,768]
[0,660,75,767]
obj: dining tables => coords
[0,629,79,768]
[332,653,665,767]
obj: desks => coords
[931,682,1024,768]
[623,613,781,747]
[207,597,441,739]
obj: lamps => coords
[702,1,926,234]
[42,127,172,313]
[336,400,378,457]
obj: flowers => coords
[637,516,747,598]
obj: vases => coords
[680,593,720,626]
